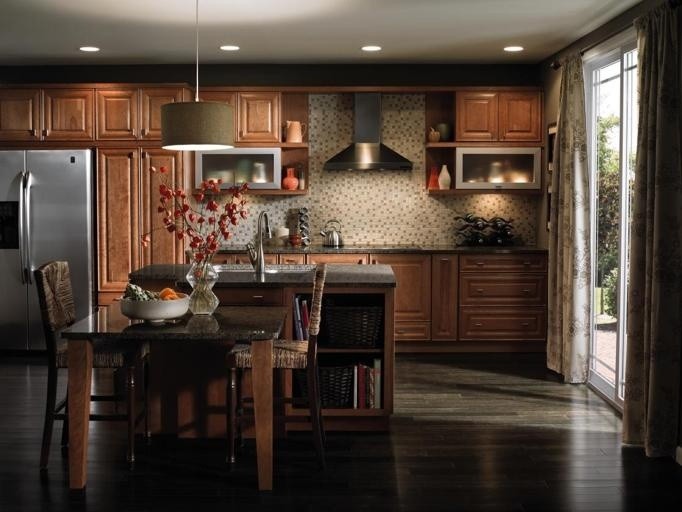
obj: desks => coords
[67,305,289,489]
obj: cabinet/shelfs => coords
[424,92,544,193]
[96,89,181,139]
[1,88,93,141]
[198,91,310,195]
[97,149,185,304]
[307,255,458,341]
[461,250,548,342]
[211,255,305,266]
[133,280,394,432]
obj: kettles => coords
[319,219,343,247]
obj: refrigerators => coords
[0,148,97,366]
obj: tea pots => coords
[285,120,307,143]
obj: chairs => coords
[226,260,329,466]
[34,259,153,473]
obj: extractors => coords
[323,142,413,171]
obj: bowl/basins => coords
[120,293,190,322]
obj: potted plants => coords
[450,215,526,249]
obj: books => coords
[353,359,381,410]
[294,296,310,340]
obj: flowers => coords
[150,164,250,256]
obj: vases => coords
[185,259,221,314]
[187,315,220,334]
